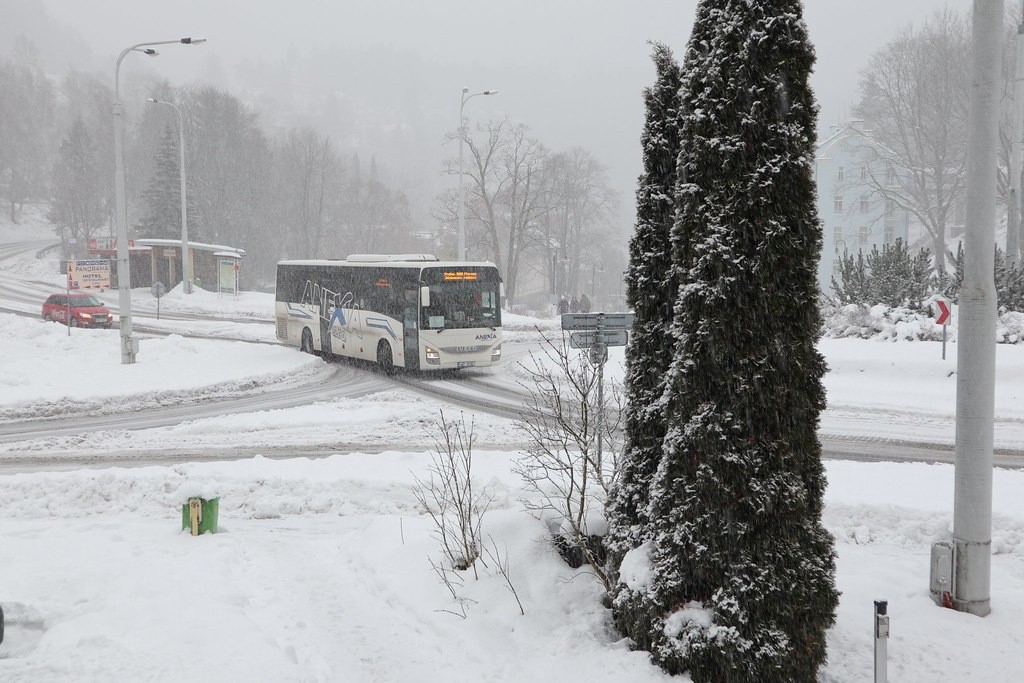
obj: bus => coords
[274,252,505,375]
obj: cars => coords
[40,290,113,329]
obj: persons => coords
[570,294,591,314]
[559,296,569,316]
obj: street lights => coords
[144,98,197,300]
[456,86,498,250]
[114,31,208,363]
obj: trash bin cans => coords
[177,479,220,535]
